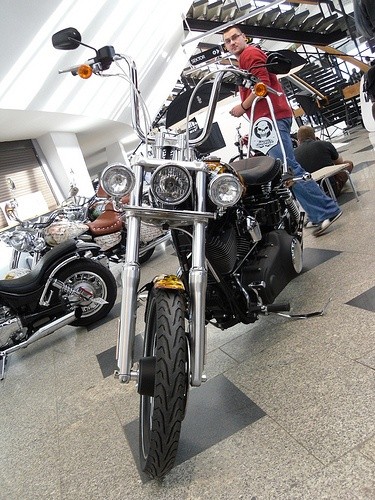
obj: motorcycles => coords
[51,26,332,481]
[0,176,171,384]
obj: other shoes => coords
[303,210,344,236]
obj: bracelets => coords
[241,102,249,110]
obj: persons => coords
[293,126,353,198]
[365,56,373,67]
[350,68,361,83]
[224,26,343,238]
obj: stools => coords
[310,164,359,203]
